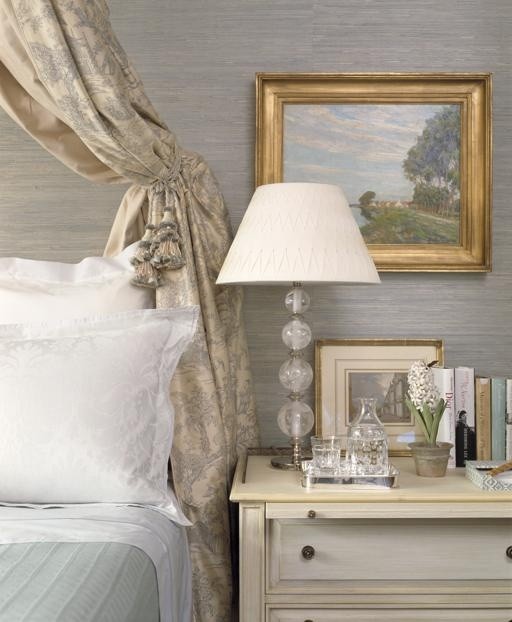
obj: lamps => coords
[213,180,384,468]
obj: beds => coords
[0,483,193,621]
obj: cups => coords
[310,426,389,478]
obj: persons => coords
[456,409,474,439]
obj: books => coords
[454,365,476,469]
[429,364,457,469]
[490,376,507,461]
[505,377,512,461]
[474,375,492,461]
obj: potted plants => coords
[402,397,454,477]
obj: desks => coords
[227,445,512,622]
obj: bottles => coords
[346,398,386,428]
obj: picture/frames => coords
[313,339,445,455]
[253,71,493,273]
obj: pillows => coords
[0,239,156,312]
[0,306,200,528]
